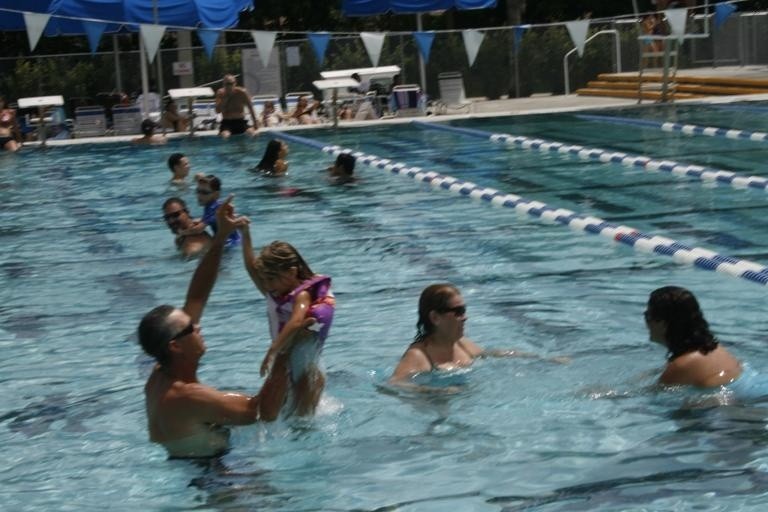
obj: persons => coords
[1,96,19,153]
[323,151,358,185]
[133,191,319,462]
[236,216,337,422]
[384,281,571,398]
[640,13,660,67]
[250,138,290,176]
[130,74,401,144]
[172,174,240,269]
[159,196,213,261]
[167,149,203,189]
[577,284,766,401]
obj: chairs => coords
[6,70,472,142]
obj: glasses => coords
[446,306,465,317]
[170,324,193,341]
[163,209,186,220]
[197,190,213,195]
